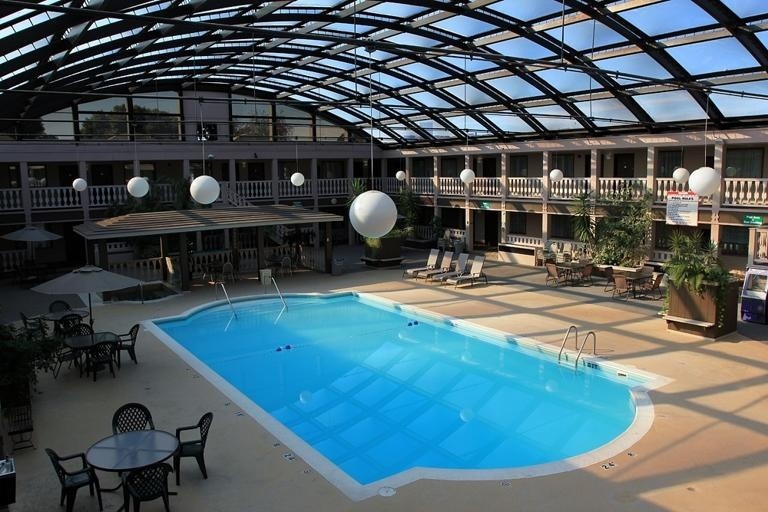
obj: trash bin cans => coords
[259,269,272,285]
[332,255,344,276]
[454,239,464,254]
[0,458,16,505]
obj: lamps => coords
[459,131,476,184]
[688,94,719,196]
[672,129,690,183]
[189,100,221,206]
[72,135,88,191]
[396,137,406,181]
[126,124,150,197]
[549,134,564,183]
[348,48,399,239]
[291,137,305,187]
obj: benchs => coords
[9,400,36,454]
[663,315,715,336]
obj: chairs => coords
[416,251,454,282]
[12,263,40,290]
[192,245,301,293]
[19,299,139,381]
[445,255,488,289]
[400,248,440,280]
[535,241,664,303]
[173,412,213,486]
[431,252,469,285]
[45,448,103,512]
[120,462,172,512]
[112,403,154,436]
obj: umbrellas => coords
[0,224,64,260]
[28,260,140,336]
